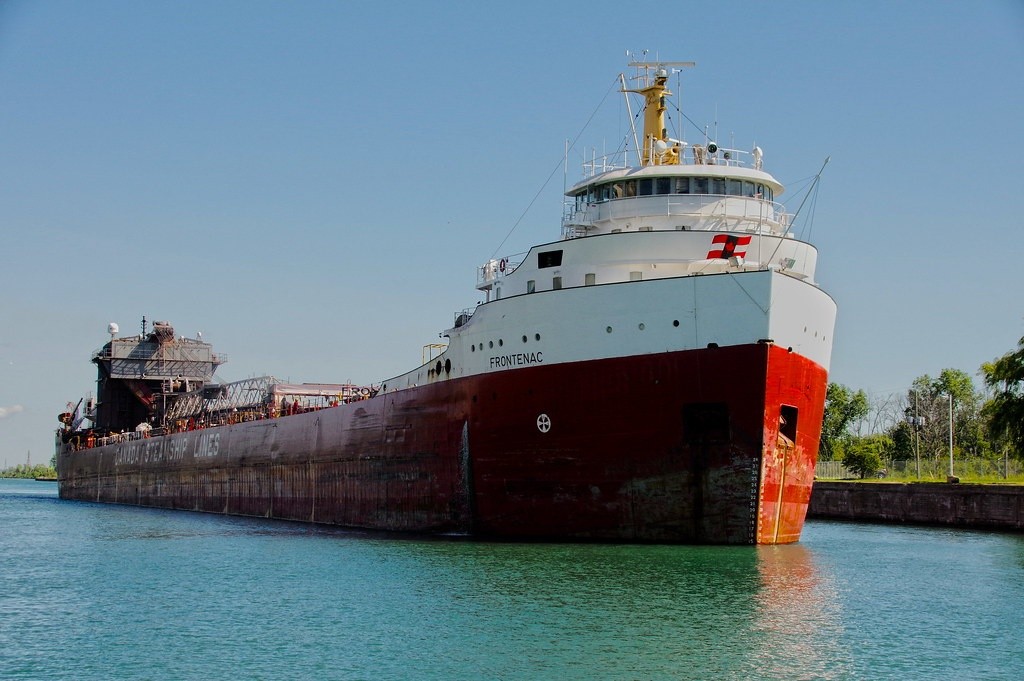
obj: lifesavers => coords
[500,259,505,272]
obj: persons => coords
[84,384,370,449]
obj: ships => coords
[52,47,838,546]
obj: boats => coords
[36,476,58,482]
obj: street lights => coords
[941,394,955,477]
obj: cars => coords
[844,468,887,479]
